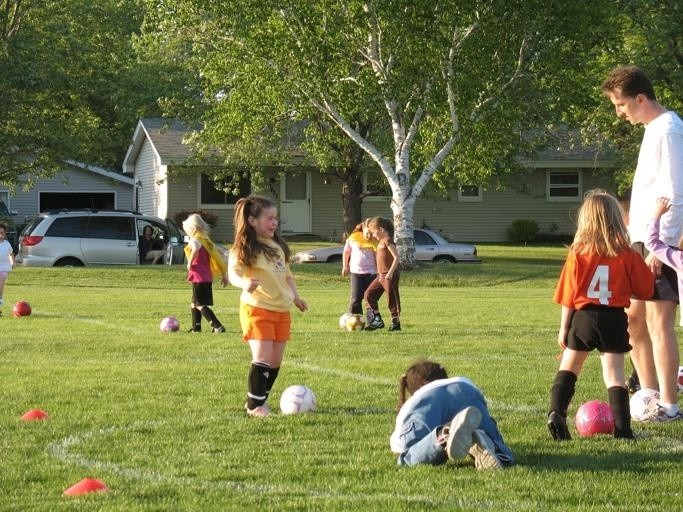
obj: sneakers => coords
[388,322,400,331]
[211,326,227,334]
[470,429,506,473]
[245,400,276,417]
[186,328,203,334]
[446,406,481,464]
[547,413,575,440]
[624,374,642,395]
[365,319,384,331]
[645,404,682,426]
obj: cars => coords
[292,225,483,266]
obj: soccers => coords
[13,301,32,317]
[339,312,351,329]
[630,388,660,422]
[676,366,683,392]
[280,385,317,415]
[576,400,614,434]
[347,313,364,331]
[160,317,180,332]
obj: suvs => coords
[0,194,19,250]
[15,205,229,267]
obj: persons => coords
[602,67,683,423]
[389,360,516,475]
[341,217,376,330]
[139,225,163,265]
[0,224,16,316]
[646,198,683,329]
[181,212,230,335]
[363,217,403,332]
[227,194,308,420]
[546,191,653,445]
